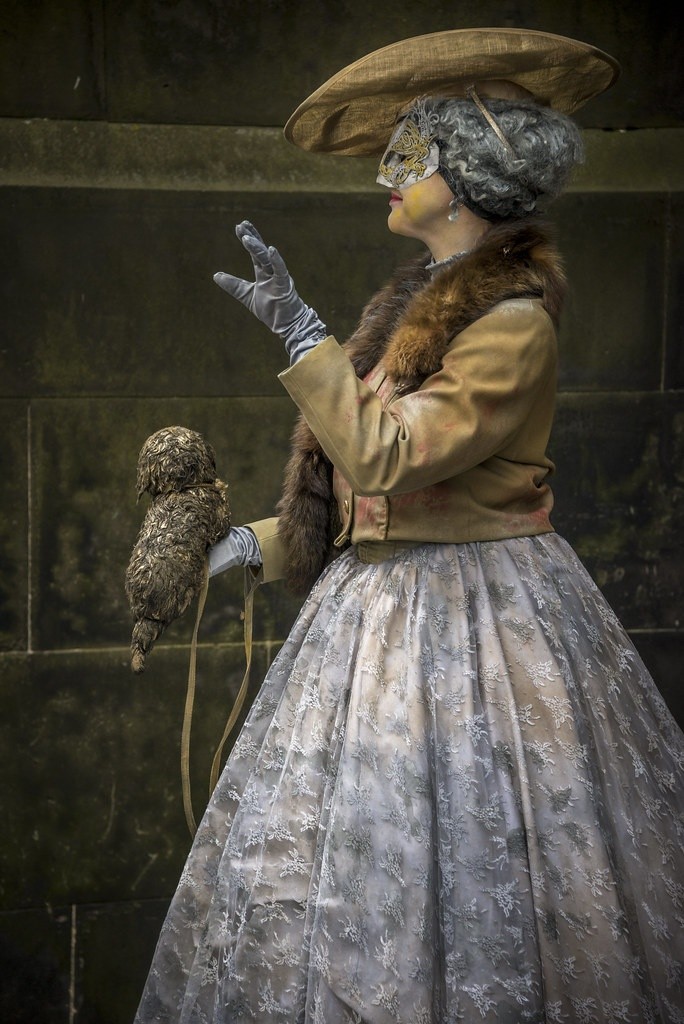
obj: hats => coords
[283,28,620,160]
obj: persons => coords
[135,28,683,1023]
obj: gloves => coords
[209,527,262,577]
[213,220,326,366]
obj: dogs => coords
[125,425,232,675]
